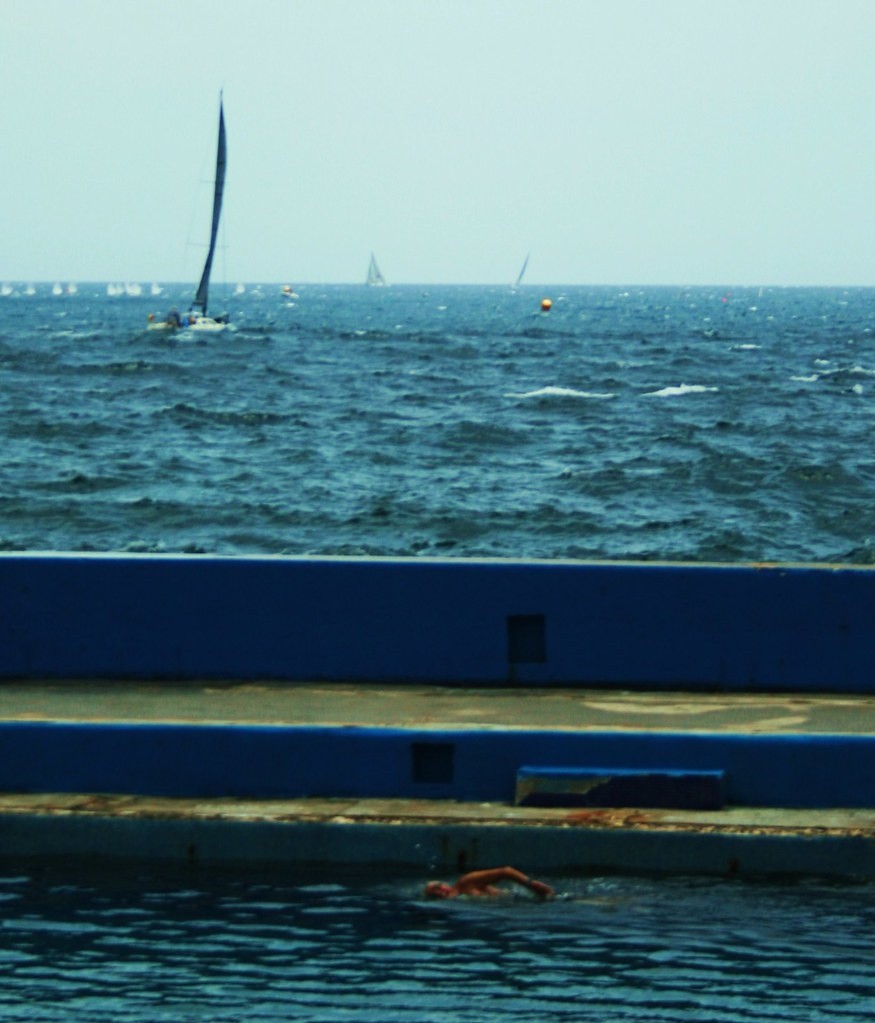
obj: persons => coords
[423,868,553,897]
[154,306,195,328]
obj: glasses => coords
[430,882,442,899]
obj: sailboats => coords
[364,252,392,288]
[0,281,249,298]
[140,87,238,337]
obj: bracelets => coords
[527,877,532,884]
